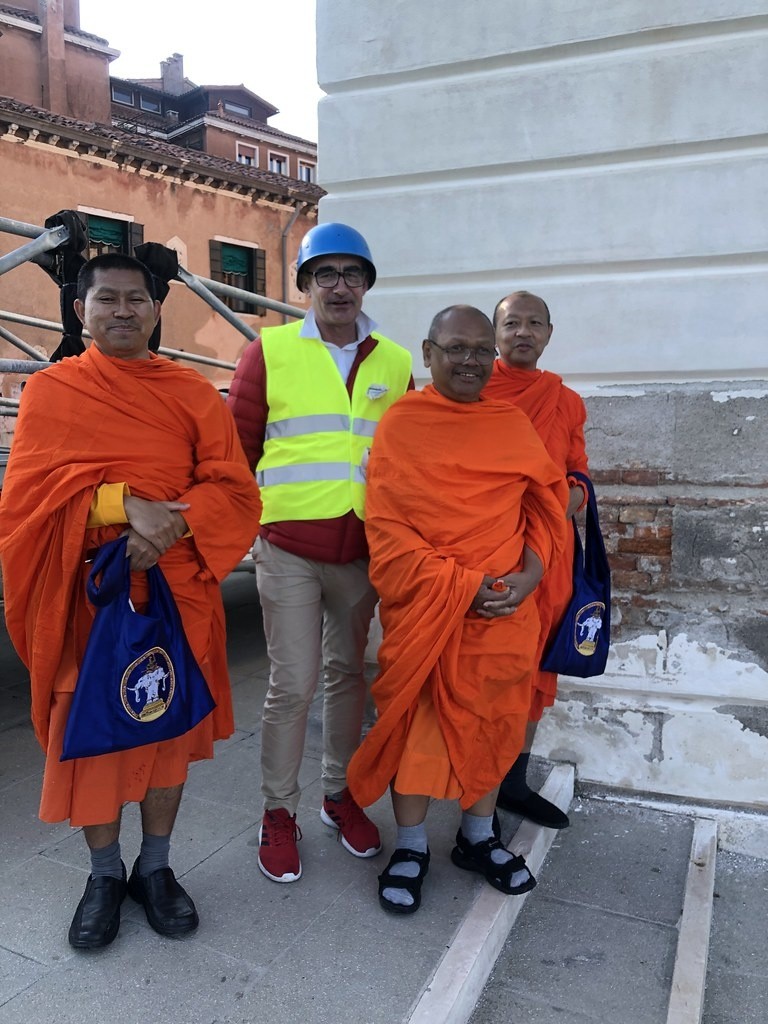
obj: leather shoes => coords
[127,857,199,939]
[67,860,128,949]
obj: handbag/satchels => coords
[56,532,222,767]
[538,466,615,679]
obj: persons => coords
[347,304,567,917]
[228,224,415,883]
[0,254,263,949]
[479,292,592,830]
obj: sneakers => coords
[257,806,304,884]
[319,790,383,858]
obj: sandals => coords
[449,828,537,896]
[378,845,432,917]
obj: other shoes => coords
[496,786,569,830]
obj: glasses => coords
[305,269,368,289]
[427,336,501,368]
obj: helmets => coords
[295,223,376,293]
